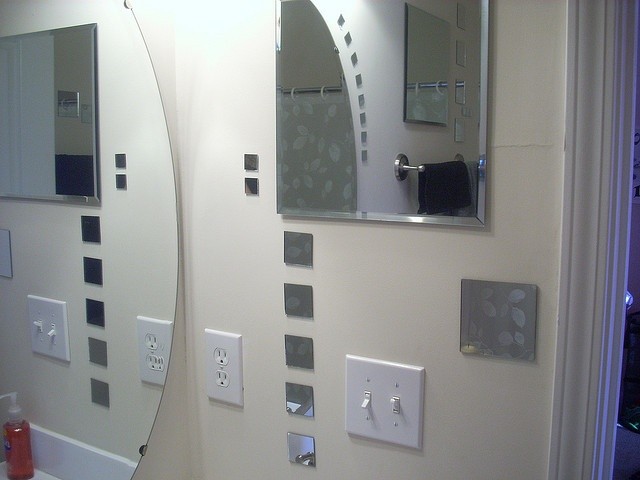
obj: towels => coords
[417,161,472,218]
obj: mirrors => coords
[276,0,358,214]
[0,0,182,478]
[404,3,450,125]
[276,2,490,231]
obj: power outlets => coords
[202,328,244,408]
[135,316,173,387]
[24,294,72,365]
[344,355,424,454]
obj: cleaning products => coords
[2,392,35,478]
